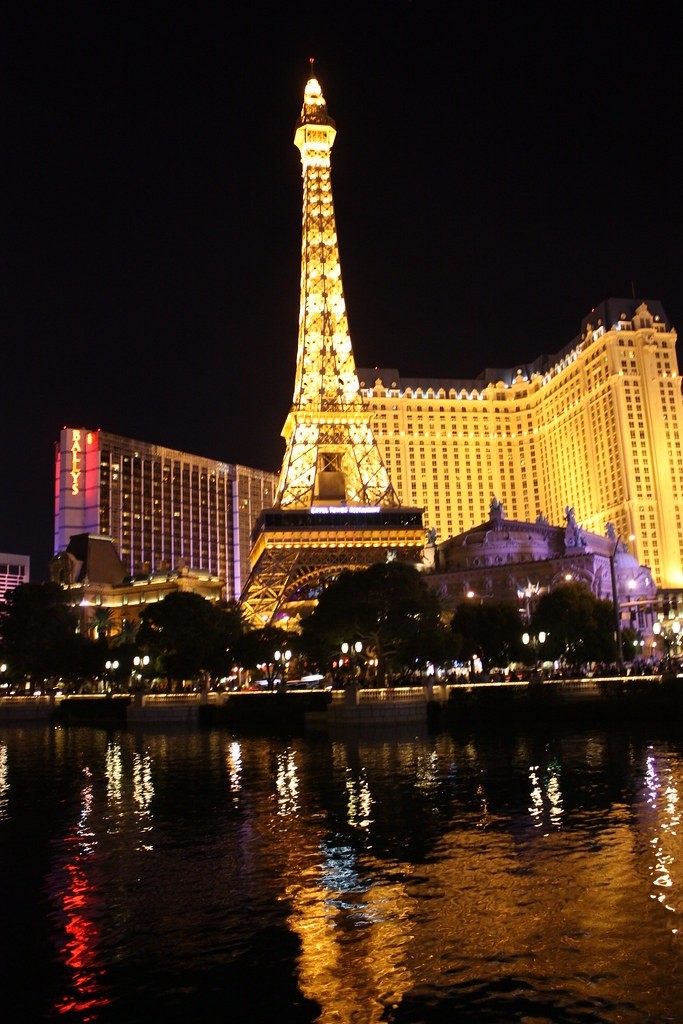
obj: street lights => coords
[134,655,150,693]
[606,532,636,675]
[522,632,546,676]
[341,641,362,687]
[105,660,120,693]
[653,621,681,673]
[275,651,292,692]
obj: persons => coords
[394,653,683,683]
